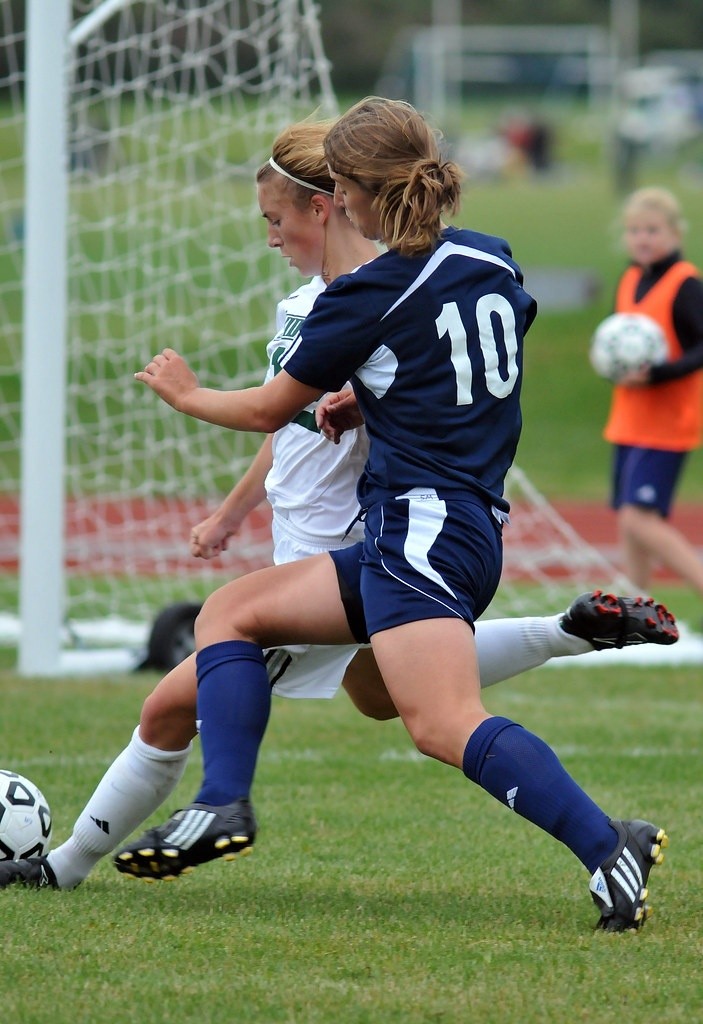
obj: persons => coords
[599,189,702,593]
[107,94,669,931]
[1,118,684,890]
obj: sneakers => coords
[0,854,80,893]
[559,589,679,650]
[113,796,258,878]
[590,819,667,934]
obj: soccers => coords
[0,768,54,863]
[588,310,669,385]
